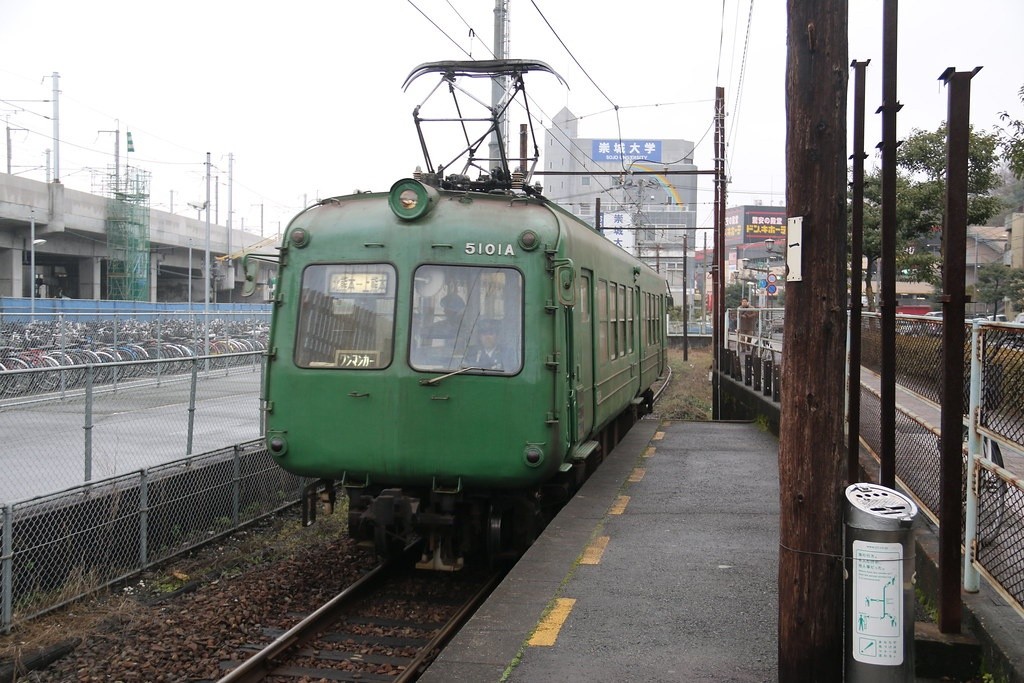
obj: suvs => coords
[925,312,943,317]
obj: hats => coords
[479,319,501,333]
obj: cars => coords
[670,322,714,334]
[965,314,1007,332]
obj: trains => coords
[258,178,674,572]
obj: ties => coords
[486,354,488,358]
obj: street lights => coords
[28,214,47,325]
[764,238,775,339]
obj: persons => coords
[737,298,758,353]
[52,287,63,298]
[460,319,519,373]
[418,293,480,365]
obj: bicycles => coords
[83,316,272,388]
[0,318,87,398]
[925,330,1023,552]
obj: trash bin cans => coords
[844,483,918,683]
